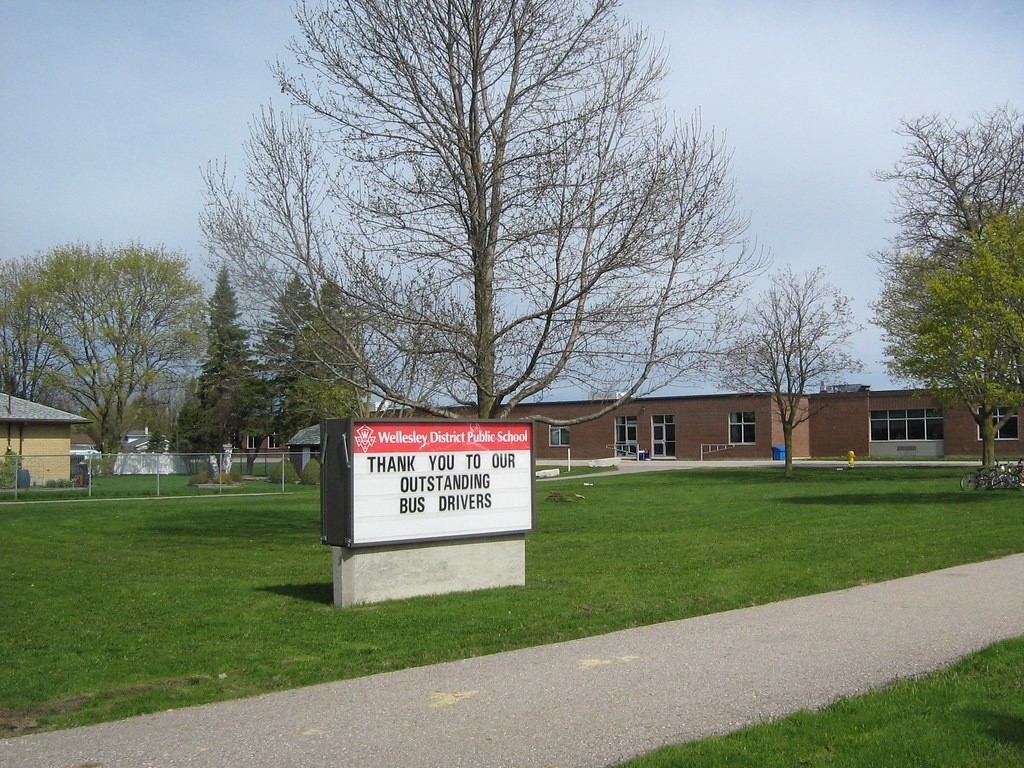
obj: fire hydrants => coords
[848,450,856,469]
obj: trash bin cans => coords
[771,446,786,460]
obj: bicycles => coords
[960,455,1024,492]
